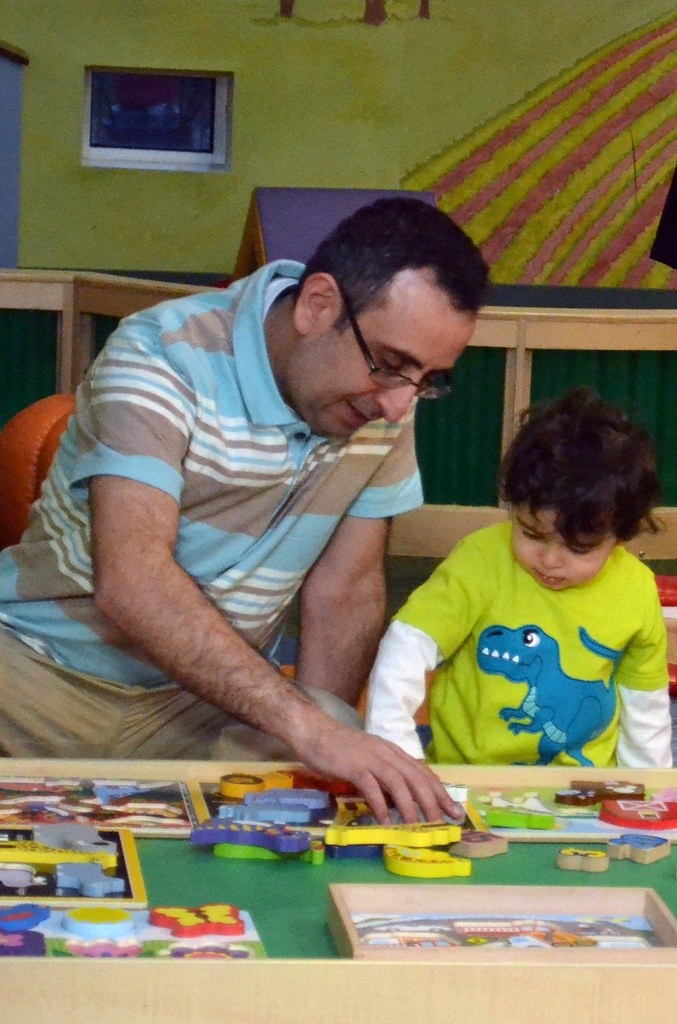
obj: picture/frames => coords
[80,64,236,174]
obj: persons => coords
[0,197,494,825]
[365,392,672,770]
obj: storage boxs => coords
[325,882,677,969]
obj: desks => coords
[0,757,677,1024]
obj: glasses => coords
[334,281,455,399]
